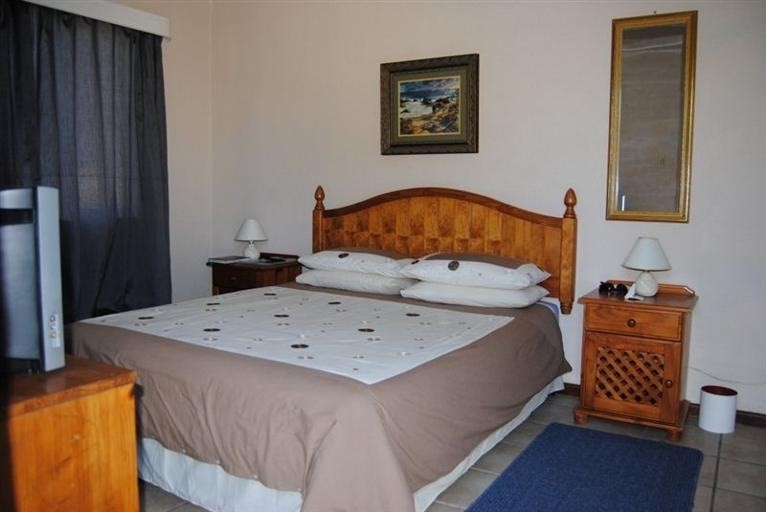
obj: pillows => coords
[291,243,555,313]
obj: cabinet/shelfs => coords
[0,347,150,510]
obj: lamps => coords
[229,216,271,262]
[621,236,672,297]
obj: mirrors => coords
[604,6,701,225]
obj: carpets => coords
[454,416,710,510]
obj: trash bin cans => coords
[698,386,738,434]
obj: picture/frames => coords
[377,53,486,157]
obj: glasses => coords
[599,282,627,295]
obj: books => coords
[208,256,250,264]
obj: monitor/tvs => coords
[0,186,66,375]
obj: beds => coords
[63,182,582,511]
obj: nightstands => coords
[202,252,304,300]
[565,274,699,441]
